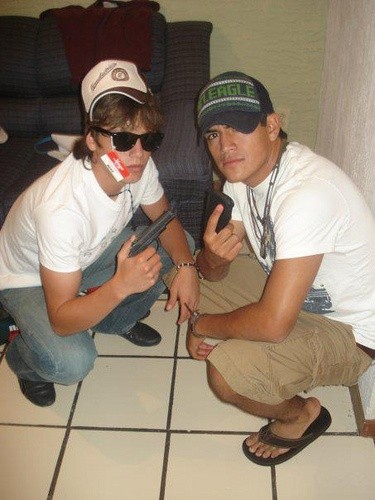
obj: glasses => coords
[91,126,165,151]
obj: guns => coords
[129,209,176,258]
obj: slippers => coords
[241,405,332,466]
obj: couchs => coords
[0,15,214,263]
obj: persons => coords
[0,58,200,406]
[186,71,374,465]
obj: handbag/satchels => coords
[32,134,83,163]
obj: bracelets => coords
[177,263,200,274]
[191,313,208,337]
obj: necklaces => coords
[246,141,289,258]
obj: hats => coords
[81,59,152,122]
[194,72,275,140]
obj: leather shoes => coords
[18,378,55,407]
[120,321,162,347]
